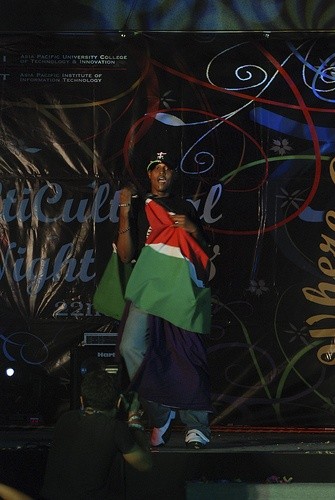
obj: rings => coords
[175,221,178,224]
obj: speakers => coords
[71,346,128,412]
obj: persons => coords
[0,369,153,500]
[114,149,210,449]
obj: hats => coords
[147,151,177,169]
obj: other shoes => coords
[128,410,143,428]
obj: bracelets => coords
[118,203,131,207]
[119,227,131,234]
[128,415,144,430]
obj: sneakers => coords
[150,410,177,450]
[184,428,210,449]
